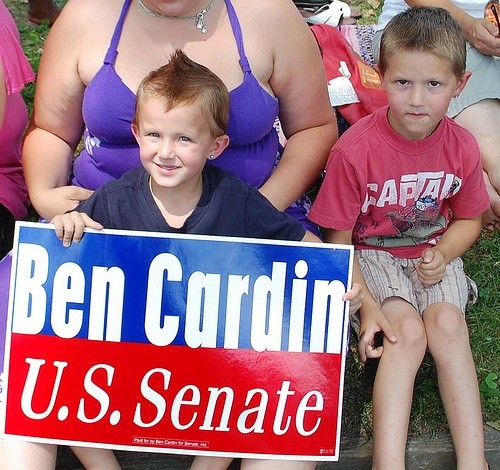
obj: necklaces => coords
[137,0,218,34]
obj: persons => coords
[0,0,38,266]
[372,0,500,231]
[32,47,366,470]
[0,0,341,470]
[305,5,492,470]
